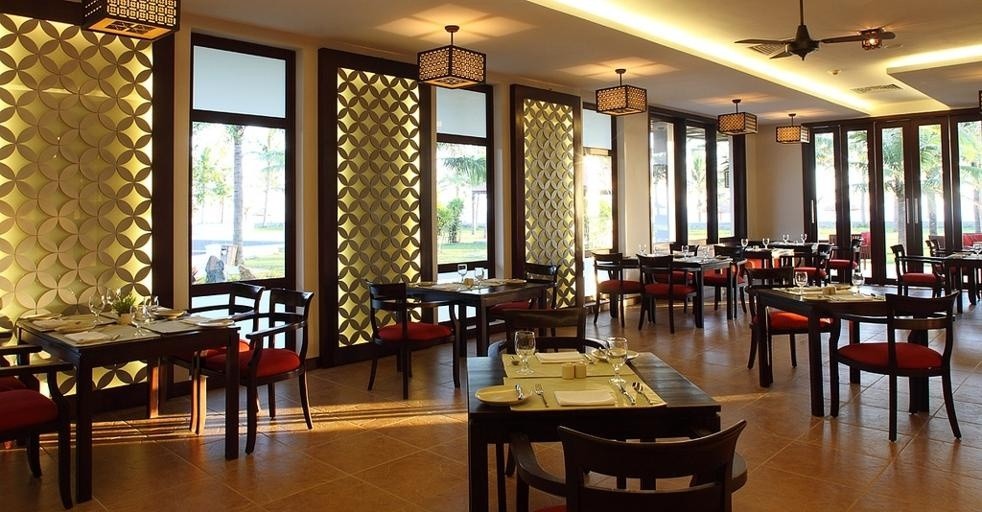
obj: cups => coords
[973,244,982,257]
[702,246,709,259]
[851,271,865,296]
[514,331,535,375]
[795,271,808,302]
[475,267,484,294]
[90,296,105,332]
[132,305,147,336]
[606,338,627,383]
[682,245,689,262]
[741,239,748,249]
[782,234,790,245]
[762,238,769,251]
[144,296,160,327]
[108,289,121,317]
[638,242,646,255]
[457,264,467,284]
[801,234,807,244]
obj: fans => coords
[734,0,896,61]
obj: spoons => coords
[632,381,653,404]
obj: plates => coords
[476,384,535,404]
[415,281,438,288]
[197,319,234,326]
[34,318,93,331]
[505,279,527,285]
[592,349,638,360]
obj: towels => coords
[64,331,113,343]
[840,295,873,300]
[535,351,584,363]
[431,283,454,289]
[554,389,615,406]
[33,319,68,329]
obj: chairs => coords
[832,291,962,442]
[780,256,827,286]
[488,308,626,512]
[0,345,73,510]
[0,332,27,448]
[487,262,562,353]
[510,420,748,512]
[930,236,946,247]
[720,237,738,273]
[367,283,461,401]
[745,268,832,383]
[745,241,767,248]
[862,232,871,258]
[853,235,862,241]
[636,254,702,334]
[742,251,780,318]
[196,288,313,454]
[891,245,945,298]
[704,246,747,318]
[655,242,699,302]
[829,235,838,244]
[158,282,266,434]
[592,253,651,328]
[926,239,970,296]
[827,239,862,286]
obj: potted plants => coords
[110,294,138,324]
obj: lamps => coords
[862,29,883,50]
[776,114,810,144]
[79,0,181,43]
[417,25,487,89]
[724,168,730,187]
[717,100,758,136]
[980,90,982,113]
[652,164,667,184]
[595,69,647,117]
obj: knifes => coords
[512,384,525,403]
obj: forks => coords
[613,378,636,407]
[534,383,547,408]
